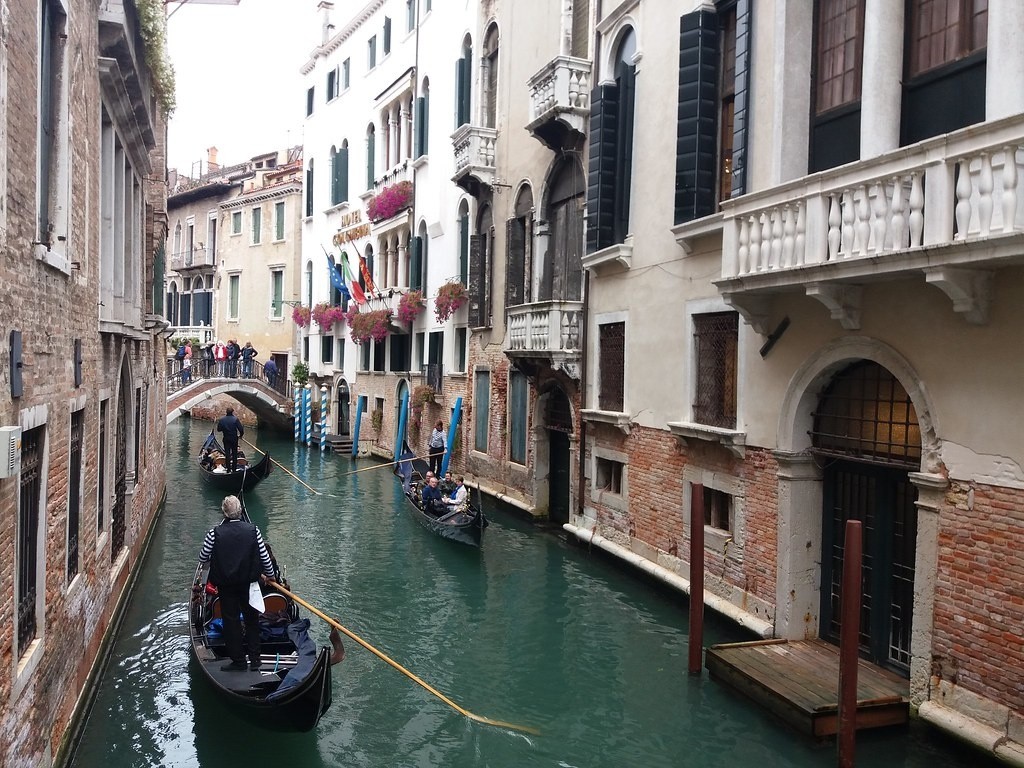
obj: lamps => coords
[271,300,302,312]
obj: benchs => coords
[313,423,331,435]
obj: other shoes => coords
[249,655,263,671]
[221,655,248,671]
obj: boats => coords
[392,438,491,549]
[196,416,275,495]
[187,469,336,732]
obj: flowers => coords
[446,425,463,464]
[433,283,469,324]
[292,307,310,328]
[284,399,295,418]
[311,399,331,421]
[366,181,415,224]
[311,302,344,333]
[346,305,395,345]
[408,383,434,449]
[398,290,426,327]
[135,0,177,120]
[371,409,383,434]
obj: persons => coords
[200,446,246,472]
[199,494,280,673]
[416,470,468,517]
[218,406,244,473]
[178,338,277,387]
[428,419,448,480]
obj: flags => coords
[357,251,378,299]
[328,258,351,300]
[341,253,367,305]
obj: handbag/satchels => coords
[202,348,212,359]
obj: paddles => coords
[261,573,472,716]
[342,451,446,475]
[241,437,319,494]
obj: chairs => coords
[264,594,290,619]
[236,458,248,465]
[213,597,223,619]
[213,457,226,468]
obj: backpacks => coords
[176,346,188,360]
